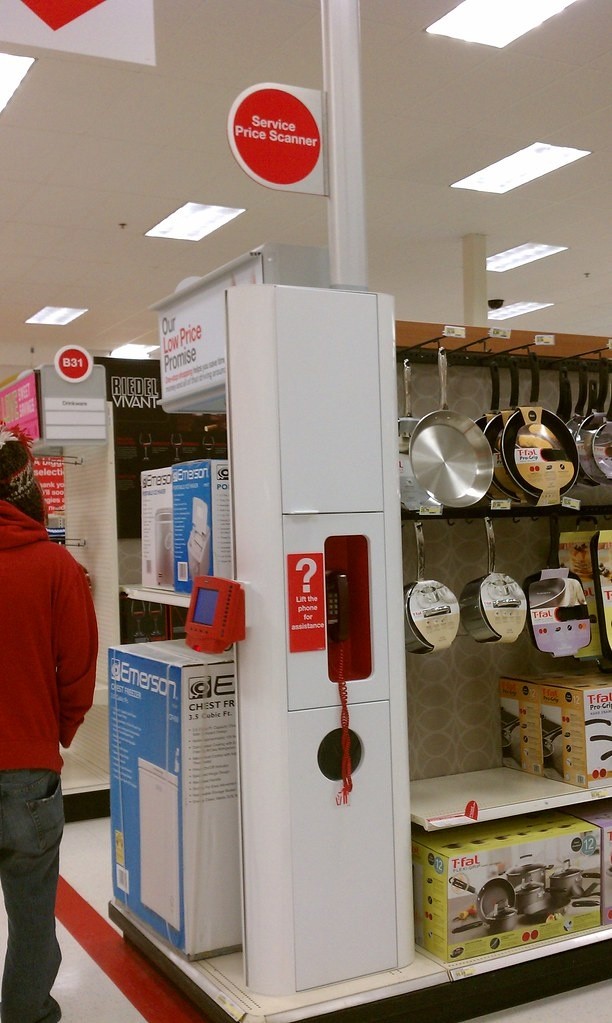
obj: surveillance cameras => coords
[488,299,503,309]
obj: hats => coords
[0,420,49,527]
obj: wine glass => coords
[171,432,182,462]
[203,436,214,459]
[139,433,151,461]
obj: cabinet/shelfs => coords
[78,324,612,1023]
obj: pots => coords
[498,854,602,920]
[452,899,519,935]
[474,347,579,505]
[398,359,444,511]
[459,517,527,644]
[523,513,592,653]
[556,352,612,487]
[449,876,516,923]
[409,347,494,508]
[403,522,460,654]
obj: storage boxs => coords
[171,458,232,603]
[140,467,173,598]
[412,808,602,962]
[560,799,612,926]
[500,671,612,790]
[107,640,244,963]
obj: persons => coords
[0,440,99,1023]
